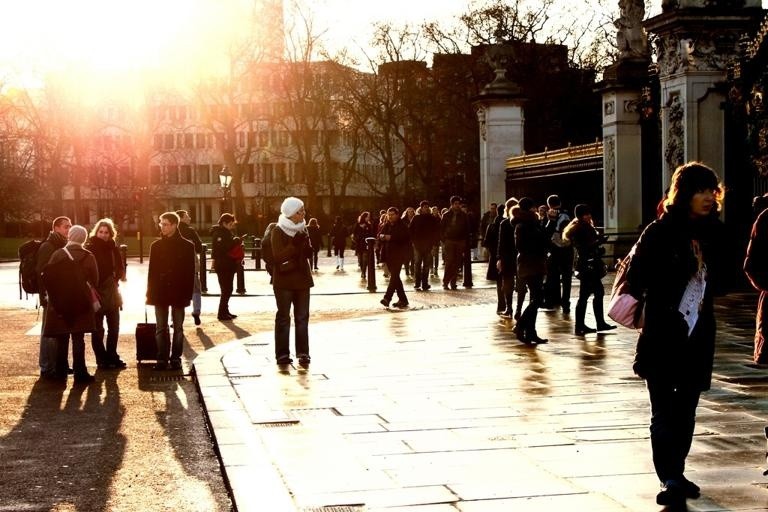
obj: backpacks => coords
[605,218,694,331]
[17,236,57,296]
[39,249,99,322]
[545,209,573,248]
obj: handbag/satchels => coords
[224,238,246,262]
[90,287,103,314]
[274,255,303,275]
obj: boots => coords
[335,255,346,273]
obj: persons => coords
[744,204,767,369]
[170,210,204,329]
[85,217,128,369]
[146,212,195,370]
[36,216,73,376]
[614,161,733,508]
[42,223,99,386]
[210,212,243,323]
[305,217,325,272]
[269,195,314,367]
[328,214,350,273]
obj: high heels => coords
[511,321,531,344]
[524,327,550,346]
[500,304,513,318]
[514,306,523,320]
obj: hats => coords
[574,204,593,217]
[518,197,537,212]
[280,195,305,219]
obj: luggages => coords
[135,300,160,365]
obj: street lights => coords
[217,164,235,214]
[252,191,264,239]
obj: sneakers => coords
[191,311,202,325]
[392,299,409,307]
[653,473,704,509]
[276,356,294,365]
[596,319,618,332]
[217,312,239,321]
[574,322,598,336]
[297,356,312,364]
[152,357,184,371]
[380,296,391,308]
[39,365,96,387]
[94,353,128,370]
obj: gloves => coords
[291,230,312,251]
